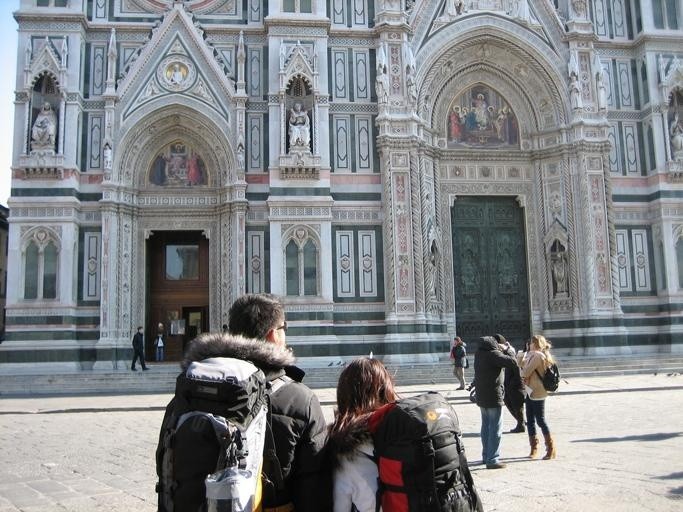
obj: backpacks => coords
[528,354,560,393]
[351,388,486,512]
[154,354,297,512]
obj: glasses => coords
[275,320,288,332]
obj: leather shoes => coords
[510,422,527,433]
[131,359,164,372]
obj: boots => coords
[543,431,558,461]
[528,433,541,458]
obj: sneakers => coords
[483,458,508,469]
[457,387,465,390]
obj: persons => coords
[519,335,556,459]
[428,253,437,300]
[597,73,607,111]
[154,332,166,362]
[474,333,509,469]
[169,64,185,86]
[450,336,467,390]
[130,325,151,372]
[330,355,403,512]
[374,64,389,104]
[30,101,59,156]
[449,91,516,145]
[288,102,313,156]
[156,292,330,512]
[151,143,202,187]
[569,73,585,110]
[553,252,568,293]
[670,114,683,164]
[494,334,526,432]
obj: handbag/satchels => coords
[464,359,469,369]
